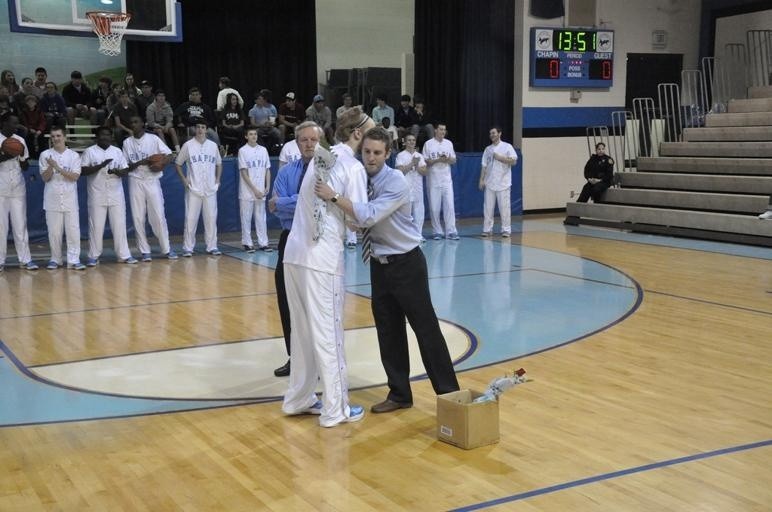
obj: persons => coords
[314,126,461,413]
[175,120,223,257]
[422,123,460,240]
[38,124,86,270]
[217,79,436,152]
[63,71,221,147]
[268,120,325,376]
[238,125,273,253]
[278,139,302,168]
[0,111,39,272]
[80,126,138,267]
[394,134,427,243]
[563,143,615,226]
[122,113,178,262]
[1,67,76,159]
[479,127,518,237]
[282,106,375,428]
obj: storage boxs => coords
[436,387,501,450]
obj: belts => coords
[369,248,419,265]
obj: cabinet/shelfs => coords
[326,65,402,129]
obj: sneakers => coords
[445,234,460,240]
[260,246,273,252]
[210,249,222,255]
[117,257,139,264]
[502,232,511,237]
[243,245,256,253]
[19,262,40,270]
[182,251,192,257]
[142,254,153,262]
[340,405,366,424]
[46,261,59,269]
[433,234,442,240]
[166,249,179,259]
[87,258,100,267]
[480,231,494,237]
[347,242,357,249]
[67,262,86,270]
[297,400,323,415]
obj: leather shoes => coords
[273,357,290,375]
[371,397,413,414]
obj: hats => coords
[313,94,326,103]
[285,92,297,101]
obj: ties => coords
[296,163,308,194]
[361,178,374,264]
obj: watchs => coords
[331,193,339,203]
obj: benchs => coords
[565,84,772,260]
[42,101,102,152]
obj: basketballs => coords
[2,138,23,158]
[149,154,164,172]
